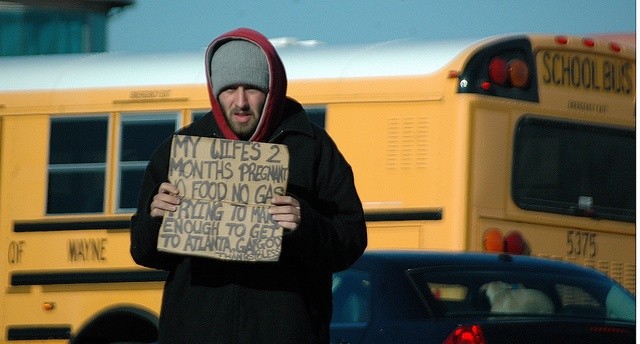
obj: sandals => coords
[0,34,636,343]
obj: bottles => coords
[209,40,269,100]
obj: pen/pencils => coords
[330,251,636,344]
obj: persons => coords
[129,27,367,344]
